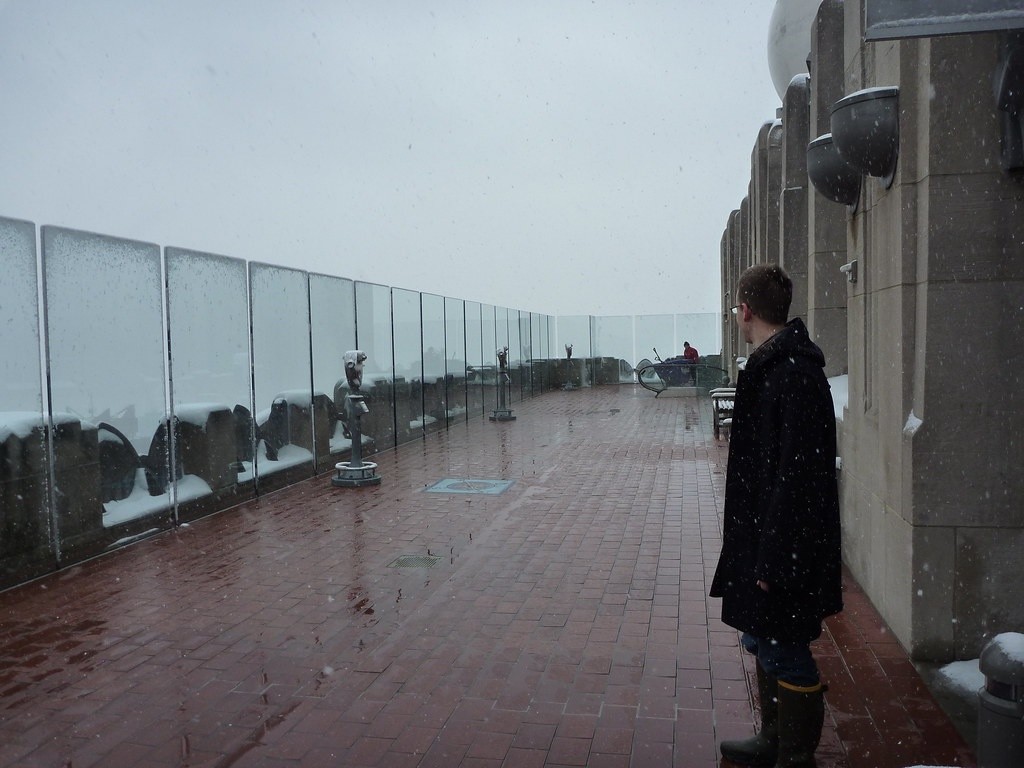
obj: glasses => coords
[730,305,744,313]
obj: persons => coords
[683,340,700,387]
[707,261,847,766]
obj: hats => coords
[684,341,689,346]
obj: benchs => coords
[709,386,736,439]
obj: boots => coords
[720,657,778,764]
[772,681,828,768]
